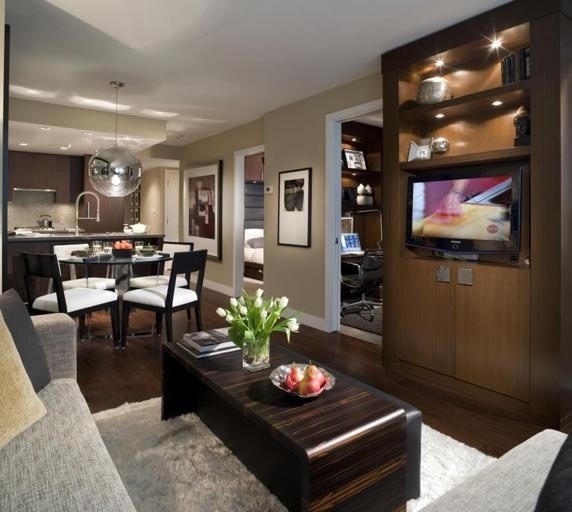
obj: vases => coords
[241,336,270,371]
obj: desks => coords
[59,248,171,338]
[9,233,165,303]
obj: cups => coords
[92,241,101,252]
[134,241,146,254]
[103,241,113,254]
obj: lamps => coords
[89,84,143,198]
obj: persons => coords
[413,179,469,223]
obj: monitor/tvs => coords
[404,166,523,266]
[341,233,361,252]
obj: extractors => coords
[13,188,57,193]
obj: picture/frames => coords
[181,158,223,262]
[278,167,311,248]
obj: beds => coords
[244,228,264,282]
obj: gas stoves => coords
[14,227,69,235]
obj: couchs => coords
[411,423,572,512]
[2,313,137,511]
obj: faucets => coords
[75,191,100,236]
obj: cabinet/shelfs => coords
[341,139,383,256]
[390,20,532,170]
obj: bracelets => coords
[448,189,463,199]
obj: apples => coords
[113,240,133,249]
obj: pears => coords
[284,358,327,394]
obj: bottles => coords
[123,223,129,233]
[356,183,374,206]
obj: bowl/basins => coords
[123,227,132,233]
[139,249,155,257]
[72,249,90,257]
[111,248,133,258]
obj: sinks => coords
[32,230,97,236]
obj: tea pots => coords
[37,214,53,227]
[129,222,150,234]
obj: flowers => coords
[215,288,300,344]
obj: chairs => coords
[48,242,115,341]
[121,249,208,349]
[129,241,192,336]
[341,249,384,322]
[20,251,120,349]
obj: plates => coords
[268,361,336,398]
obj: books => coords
[500,47,531,85]
[175,327,242,359]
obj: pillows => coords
[1,289,49,391]
[1,312,47,448]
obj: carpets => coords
[92,390,498,512]
